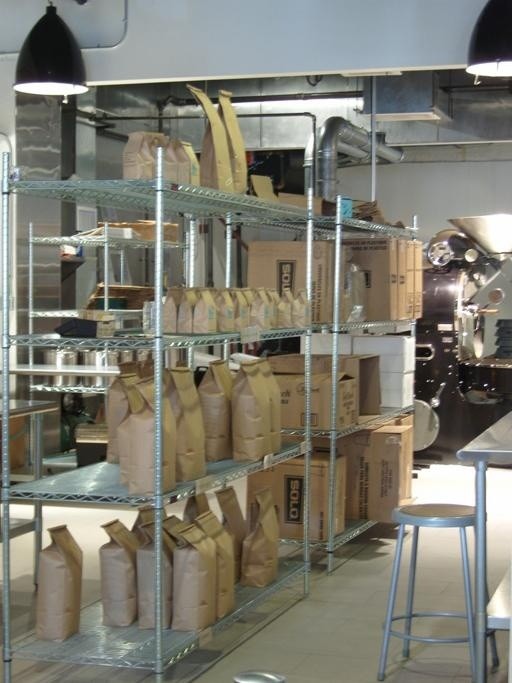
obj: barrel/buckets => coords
[43,339,230,387]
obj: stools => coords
[378,502,500,682]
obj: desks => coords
[456,406,512,681]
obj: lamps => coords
[13,0,89,96]
[464,0,512,76]
